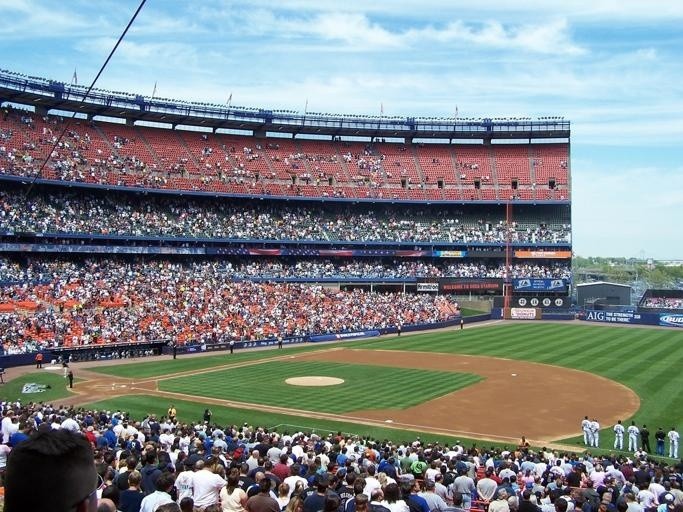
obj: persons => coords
[66,369,73,389]
[0,399,682,512]
[1,102,572,367]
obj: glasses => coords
[73,473,104,507]
